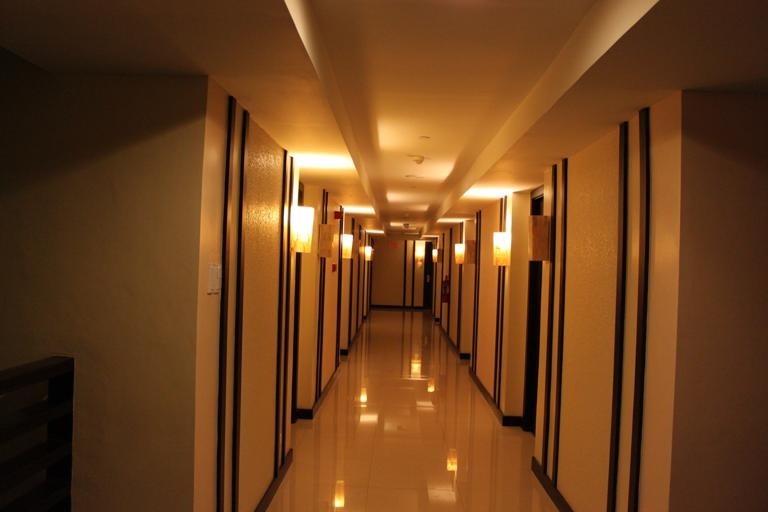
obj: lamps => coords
[290,205,372,263]
[431,215,551,266]
